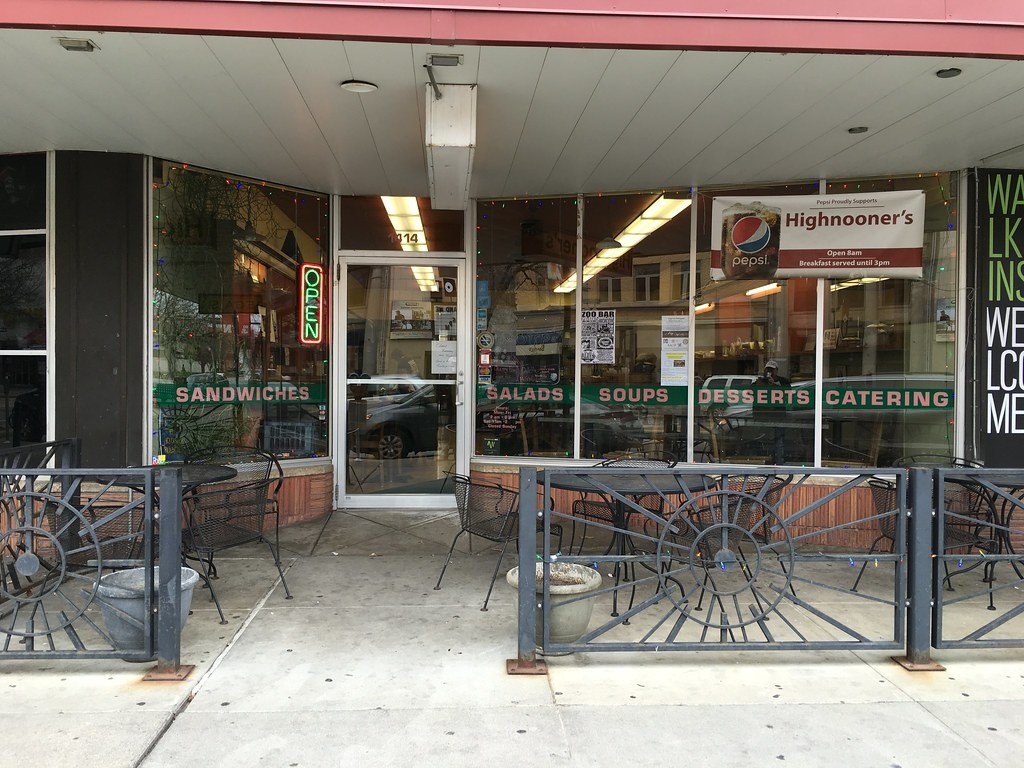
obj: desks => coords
[95,463,238,589]
[537,470,716,618]
[631,432,737,463]
[944,474,1024,582]
[538,411,633,457]
[729,410,830,466]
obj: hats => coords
[765,361,778,369]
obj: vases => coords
[505,561,602,658]
[92,564,201,662]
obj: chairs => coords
[433,470,563,612]
[849,454,1003,611]
[579,429,766,461]
[568,449,797,626]
[18,445,294,645]
[602,408,665,462]
[708,403,770,464]
[518,402,574,458]
[821,411,885,468]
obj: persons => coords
[750,361,792,465]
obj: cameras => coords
[761,372,774,385]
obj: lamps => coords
[381,196,439,293]
[596,198,622,249]
[695,302,716,316]
[746,281,787,300]
[230,185,266,243]
[552,192,692,294]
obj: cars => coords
[186,372,230,388]
[261,371,958,465]
[247,368,292,386]
